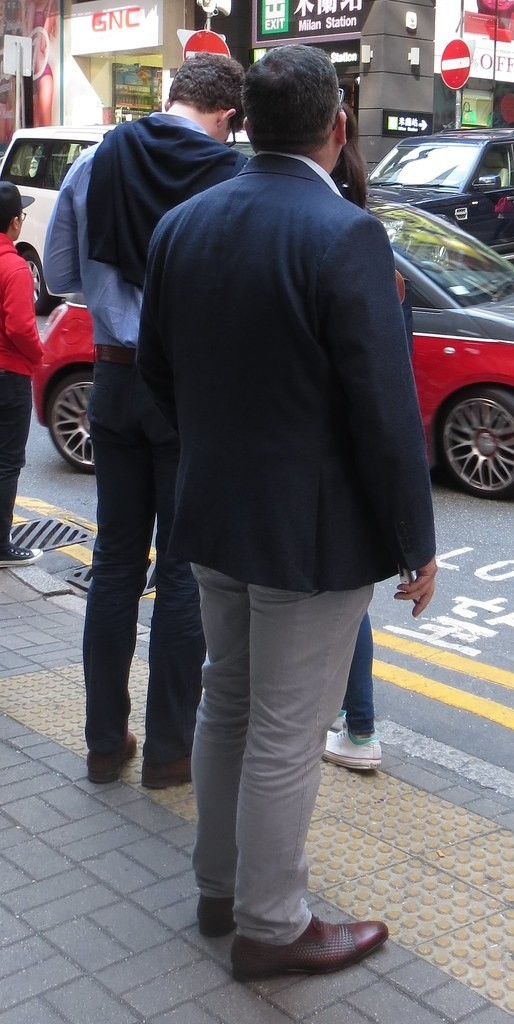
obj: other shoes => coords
[87,732,137,783]
[141,751,191,787]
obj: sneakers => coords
[329,710,348,730]
[0,543,43,568]
[320,723,382,769]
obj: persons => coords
[141,44,438,977]
[43,54,380,790]
[0,182,44,568]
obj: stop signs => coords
[182,30,231,64]
[440,39,472,90]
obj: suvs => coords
[0,123,258,318]
[359,121,514,265]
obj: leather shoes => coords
[230,914,389,980]
[197,894,236,937]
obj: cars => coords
[32,194,514,501]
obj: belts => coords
[96,345,137,367]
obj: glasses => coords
[19,211,27,222]
[224,118,236,147]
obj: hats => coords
[0,181,35,219]
